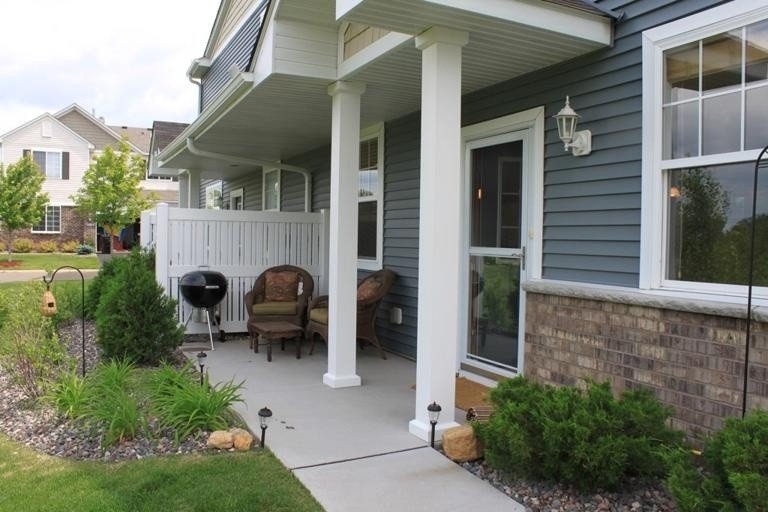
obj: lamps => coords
[427,401,442,448]
[196,349,208,385]
[552,95,591,157]
[259,407,272,448]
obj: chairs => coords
[246,264,314,350]
[307,269,394,361]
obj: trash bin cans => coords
[101,237,112,252]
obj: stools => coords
[250,321,303,362]
